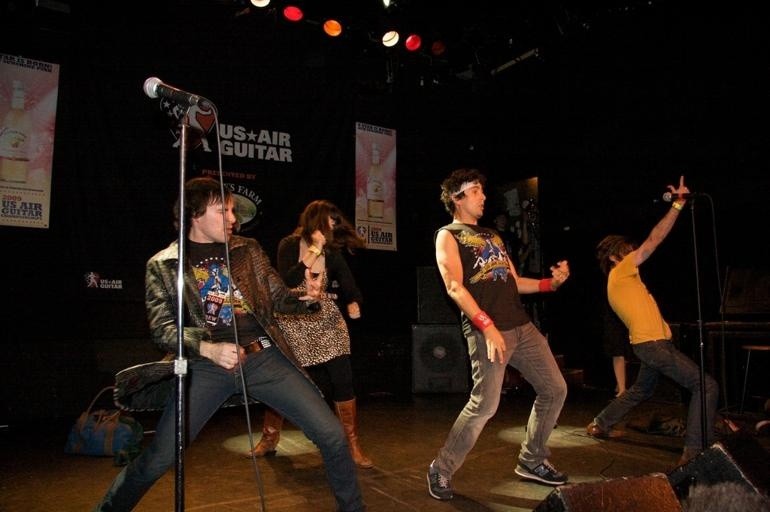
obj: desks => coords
[690,322,770,418]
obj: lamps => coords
[249,0,448,58]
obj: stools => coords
[740,345,769,417]
[734,344,770,413]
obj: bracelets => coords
[472,309,494,331]
[308,243,321,256]
[672,200,683,211]
[540,277,556,294]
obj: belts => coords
[241,335,272,356]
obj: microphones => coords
[663,192,706,203]
[144,76,211,109]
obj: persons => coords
[425,165,572,501]
[246,200,375,470]
[94,177,369,512]
[492,207,530,269]
[587,175,728,512]
[594,275,631,398]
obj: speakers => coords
[409,324,470,394]
[414,265,461,324]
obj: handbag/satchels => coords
[65,385,145,466]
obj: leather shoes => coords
[677,456,688,466]
[585,421,609,438]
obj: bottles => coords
[0,79,32,183]
[367,143,385,218]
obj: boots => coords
[332,395,374,470]
[246,409,283,459]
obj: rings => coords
[556,269,563,277]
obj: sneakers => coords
[514,456,569,485]
[425,458,455,501]
